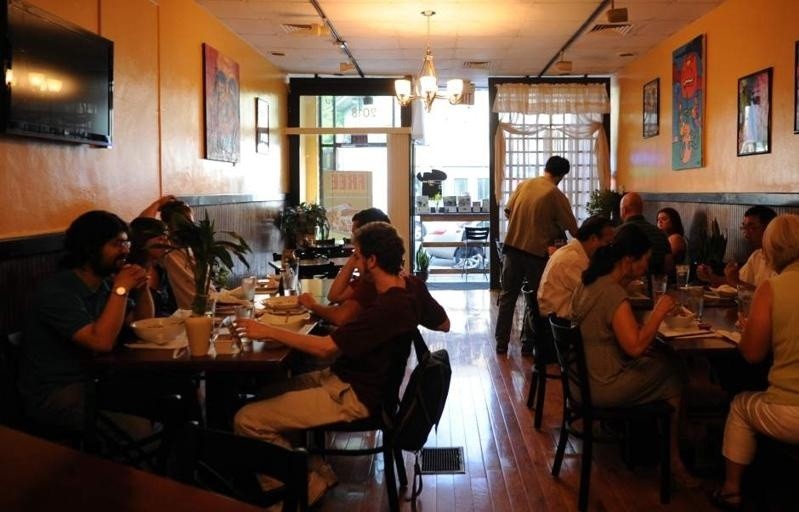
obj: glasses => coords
[105,236,130,252]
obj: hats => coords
[130,217,165,237]
[63,210,131,263]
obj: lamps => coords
[311,18,330,36]
[555,51,572,72]
[606,0,628,22]
[394,9,464,114]
[340,56,356,73]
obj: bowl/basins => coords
[709,285,737,299]
[254,313,309,338]
[661,305,697,330]
[128,317,187,345]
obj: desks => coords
[414,212,490,274]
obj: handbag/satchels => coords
[379,326,451,450]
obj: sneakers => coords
[269,463,340,511]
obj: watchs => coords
[110,285,129,298]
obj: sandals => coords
[705,483,743,509]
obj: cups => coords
[676,264,691,287]
[239,275,257,303]
[234,304,254,344]
[184,313,212,357]
[683,285,705,322]
[736,284,751,332]
[650,274,668,303]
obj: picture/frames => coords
[256,97,269,153]
[792,40,799,134]
[641,77,659,139]
[736,67,772,156]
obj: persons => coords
[133,194,217,311]
[298,268,452,333]
[495,156,577,356]
[122,217,180,332]
[229,218,420,510]
[327,207,395,303]
[14,208,202,461]
[535,192,799,510]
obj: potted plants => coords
[416,244,433,282]
[272,202,330,246]
[141,208,254,358]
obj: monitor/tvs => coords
[0,0,114,146]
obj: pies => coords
[262,296,308,314]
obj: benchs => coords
[290,326,440,512]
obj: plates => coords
[261,297,300,309]
[252,281,278,290]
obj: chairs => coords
[520,281,563,429]
[545,311,677,512]
[272,237,352,279]
[494,239,504,306]
[460,226,488,282]
[753,432,799,512]
[166,418,309,512]
[0,325,25,430]
